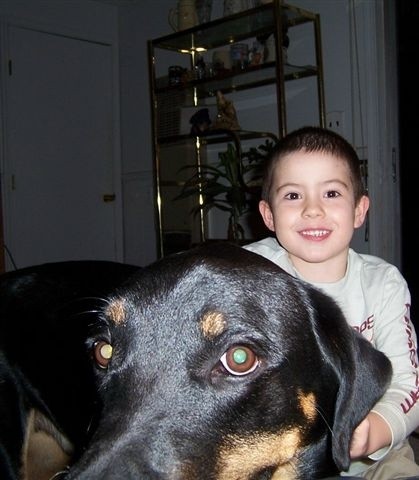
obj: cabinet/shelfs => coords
[145,0,328,261]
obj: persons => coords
[240,126,419,480]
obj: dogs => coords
[0,244,393,480]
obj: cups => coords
[168,0,196,32]
